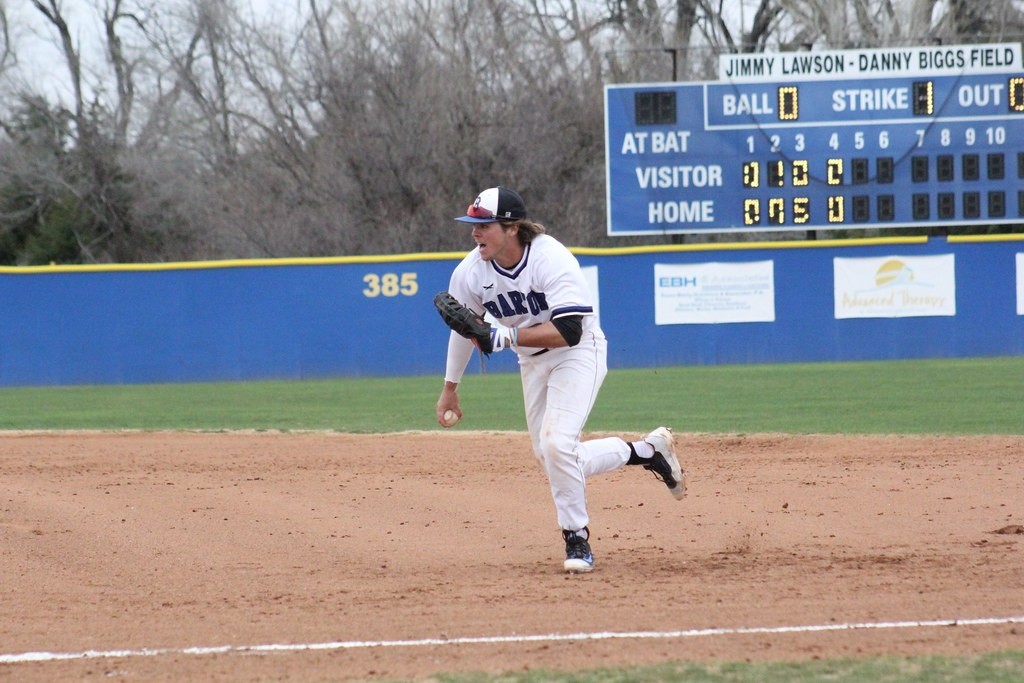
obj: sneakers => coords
[563,536,593,572]
[643,427,686,501]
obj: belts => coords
[532,348,549,356]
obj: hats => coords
[454,186,527,223]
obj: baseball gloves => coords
[433,292,492,354]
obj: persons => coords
[436,186,687,574]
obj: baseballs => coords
[444,410,459,427]
